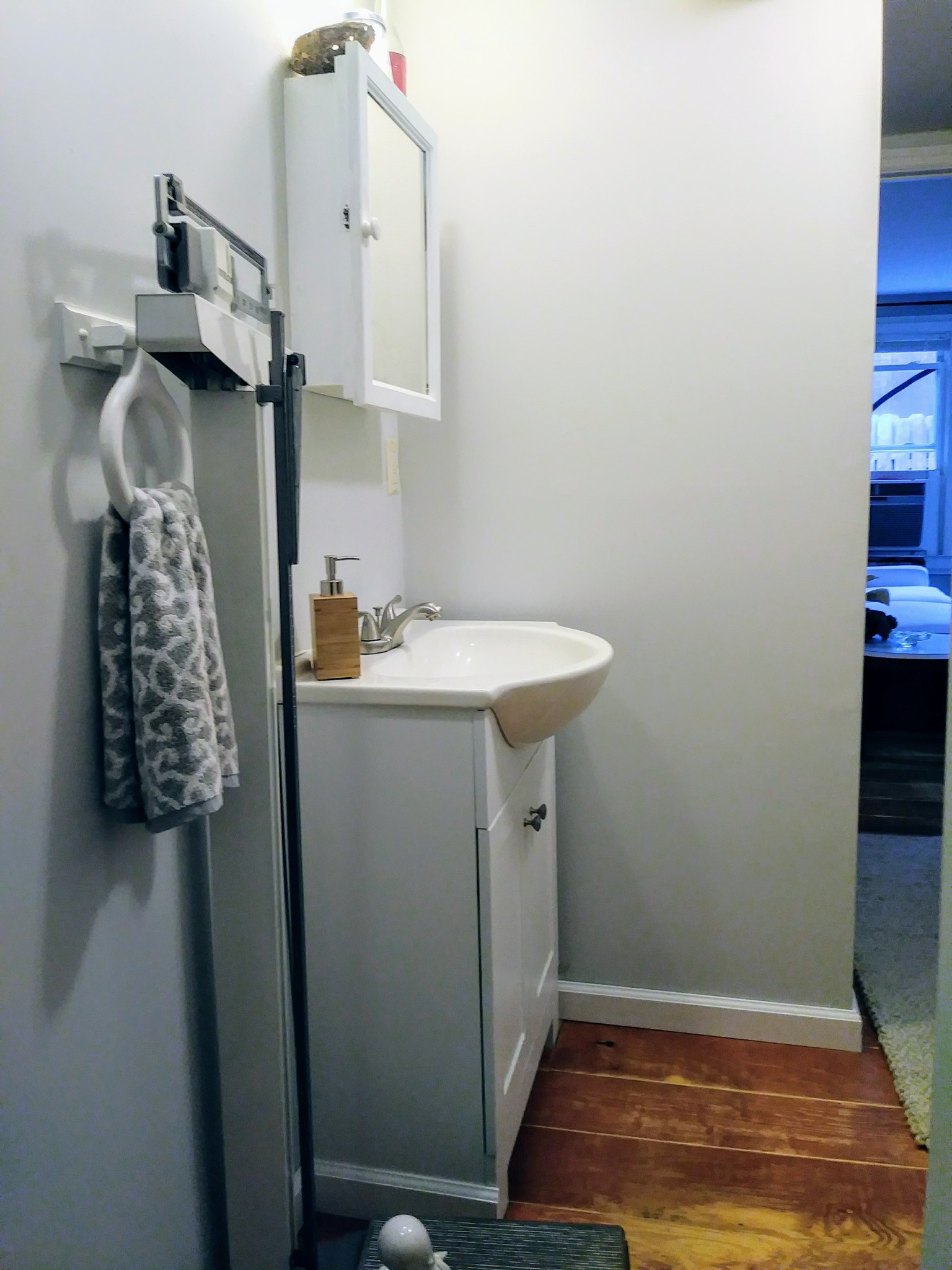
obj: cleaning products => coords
[308,555,361,681]
[371,0,407,99]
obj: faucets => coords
[358,593,442,655]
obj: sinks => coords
[372,620,614,749]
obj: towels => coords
[96,479,243,835]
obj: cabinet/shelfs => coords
[285,39,442,421]
[276,701,562,1225]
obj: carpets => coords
[853,832,942,1147]
